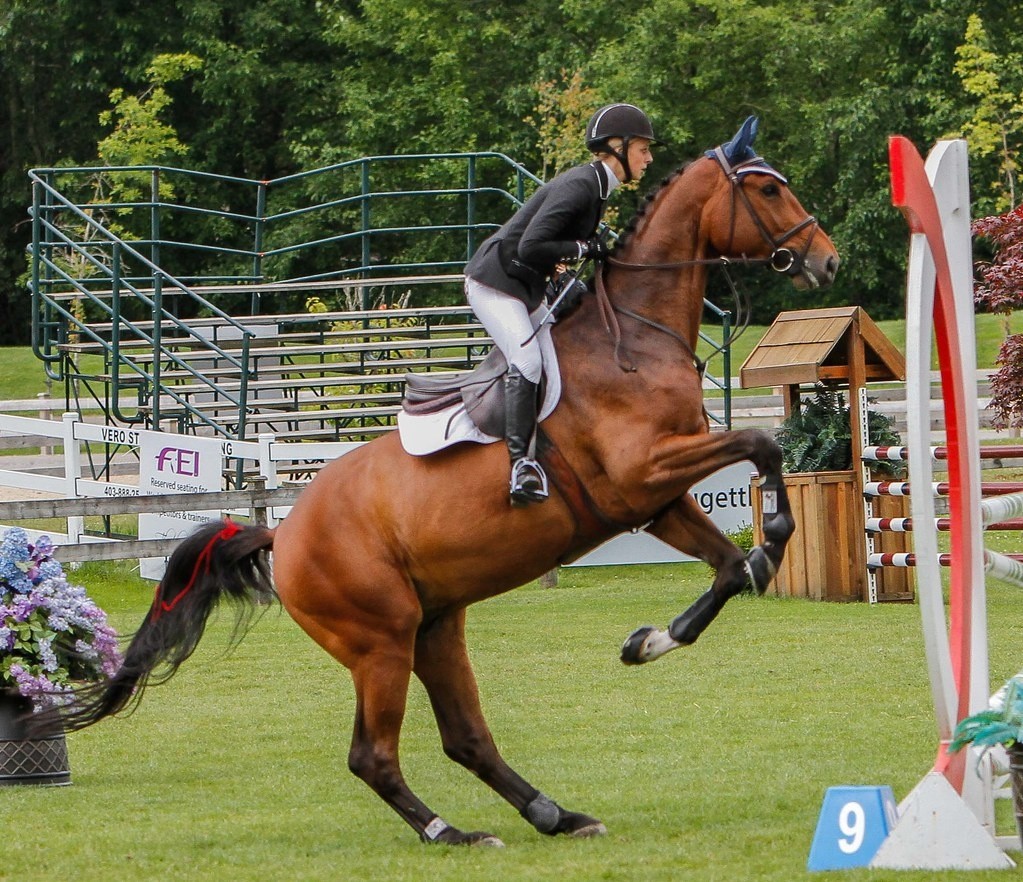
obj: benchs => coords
[40,272,499,476]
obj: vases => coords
[0,694,73,786]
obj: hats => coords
[585,100,665,147]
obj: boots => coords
[501,368,543,496]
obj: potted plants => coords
[946,677,1023,853]
[738,305,915,605]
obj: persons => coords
[543,261,589,317]
[459,103,662,504]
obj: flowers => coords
[0,527,137,714]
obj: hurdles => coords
[857,388,1022,603]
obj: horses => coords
[0,114,840,851]
